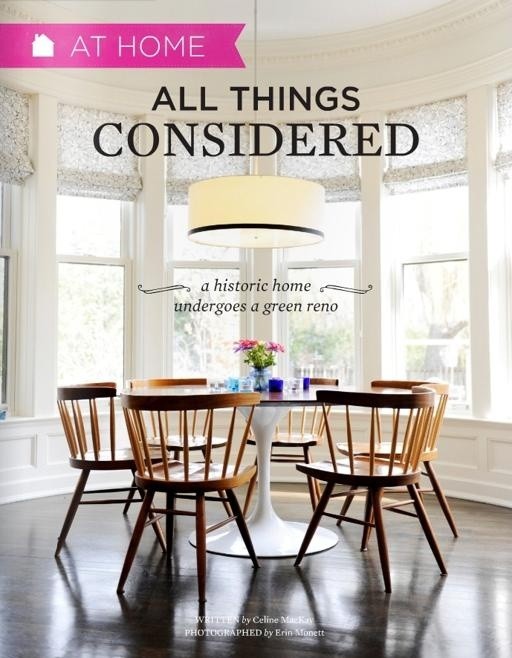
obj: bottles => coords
[241,367,284,392]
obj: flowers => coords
[232,340,285,387]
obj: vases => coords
[247,369,271,393]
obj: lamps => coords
[187,1,329,248]
